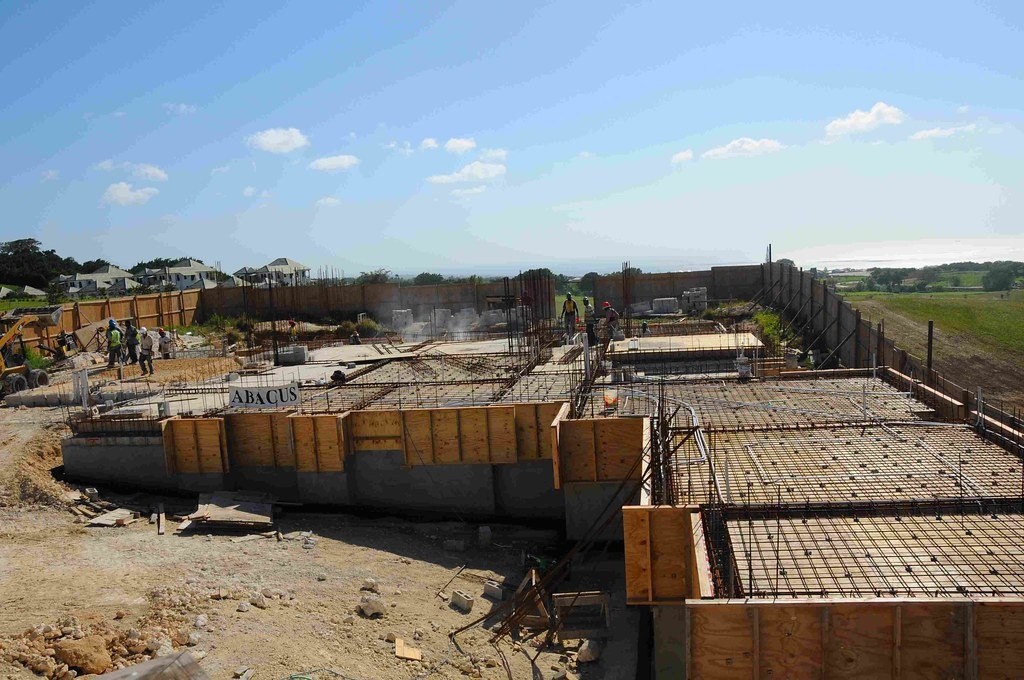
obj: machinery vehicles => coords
[0,304,63,394]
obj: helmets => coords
[140,327,147,334]
[108,320,116,326]
[576,317,581,324]
[583,297,588,301]
[158,328,164,333]
[601,301,611,310]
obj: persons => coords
[107,322,122,367]
[138,327,155,376]
[158,328,173,359]
[583,297,597,346]
[560,291,579,337]
[124,320,140,363]
[286,320,297,344]
[602,300,620,326]
[348,330,362,345]
[106,319,125,341]
[639,322,651,337]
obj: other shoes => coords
[148,373,155,377]
[129,361,136,364]
[107,364,114,368]
[141,371,148,376]
